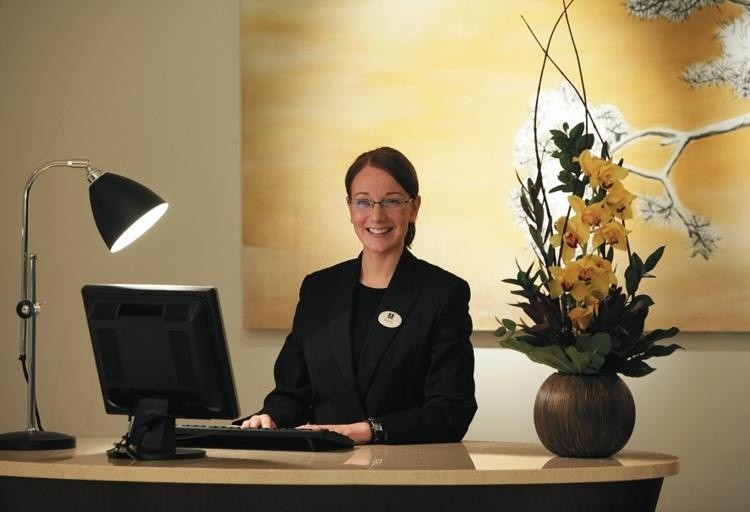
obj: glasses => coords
[349,197,413,210]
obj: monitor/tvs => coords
[80,283,242,460]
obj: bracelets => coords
[362,417,384,445]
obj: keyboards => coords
[174,424,355,452]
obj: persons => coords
[232,146,478,445]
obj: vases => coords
[533,373,636,458]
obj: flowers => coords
[497,0,685,373]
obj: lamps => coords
[0,160,169,450]
